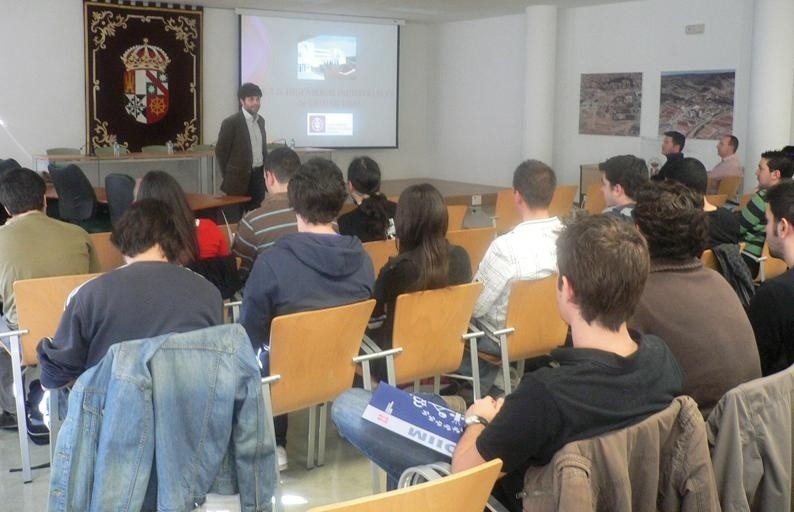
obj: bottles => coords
[114,142,120,158]
[290,137,296,149]
[167,140,173,154]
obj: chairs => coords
[47,142,288,155]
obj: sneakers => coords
[28,379,44,405]
[275,445,289,472]
[0,414,17,428]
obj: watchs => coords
[463,414,490,432]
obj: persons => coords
[745,179,794,376]
[708,133,744,196]
[357,183,475,382]
[627,178,764,421]
[665,157,741,251]
[466,158,569,358]
[737,150,794,280]
[0,142,398,473]
[215,82,269,213]
[329,212,688,512]
[650,130,685,181]
[598,154,650,219]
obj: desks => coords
[32,146,335,196]
[380,177,512,208]
